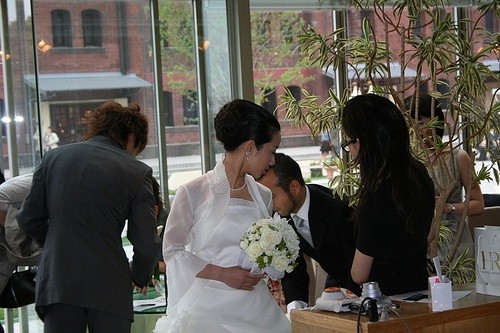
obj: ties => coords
[292,216,313,247]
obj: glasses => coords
[341,139,356,151]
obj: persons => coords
[340,93,436,298]
[17,99,162,333]
[144,175,178,280]
[252,149,372,313]
[46,126,59,151]
[0,168,58,333]
[150,98,302,332]
[401,92,485,288]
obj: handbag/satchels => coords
[0,268,39,307]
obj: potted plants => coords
[325,158,338,179]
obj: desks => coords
[290,282,500,333]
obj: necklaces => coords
[226,179,247,194]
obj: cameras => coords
[350,281,394,315]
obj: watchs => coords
[448,202,455,214]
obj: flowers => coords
[238,212,301,280]
